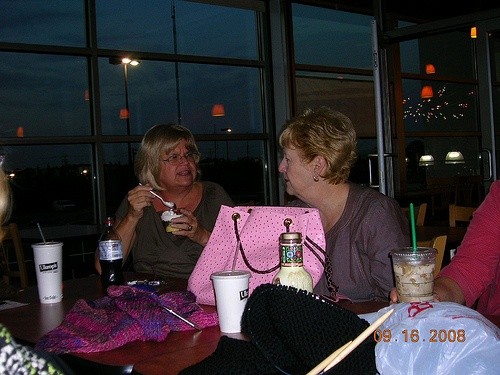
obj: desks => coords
[0,272,389,375]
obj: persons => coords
[276,103,410,303]
[0,166,64,375]
[94,121,237,282]
[388,178,500,330]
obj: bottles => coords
[98,216,123,288]
[271,232,313,293]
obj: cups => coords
[32,242,63,303]
[210,271,251,331]
[162,214,187,241]
[390,247,438,303]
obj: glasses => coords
[160,152,200,165]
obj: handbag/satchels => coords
[186,205,326,305]
[358,302,500,375]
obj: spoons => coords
[139,182,174,208]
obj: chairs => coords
[401,202,478,278]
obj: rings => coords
[188,224,192,231]
